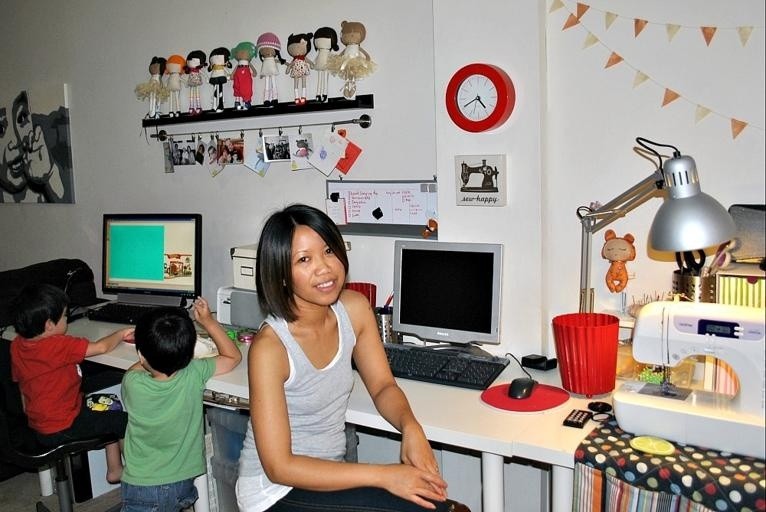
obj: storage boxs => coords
[229,291,266,329]
[204,408,360,512]
[230,244,260,292]
[215,286,234,325]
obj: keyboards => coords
[351,346,510,390]
[89,303,159,325]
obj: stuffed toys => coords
[601,228,636,294]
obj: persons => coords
[328,21,377,99]
[0,86,72,205]
[311,26,338,103]
[196,144,204,165]
[172,144,181,165]
[207,145,217,161]
[181,147,188,164]
[284,31,315,105]
[119,296,242,512]
[234,203,471,511]
[182,50,208,114]
[258,34,287,107]
[164,55,185,118]
[230,40,258,110]
[217,138,241,163]
[187,145,195,165]
[135,57,167,118]
[265,141,290,160]
[8,283,137,485]
[206,46,232,113]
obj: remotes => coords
[562,409,592,430]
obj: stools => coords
[0,438,118,512]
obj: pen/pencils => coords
[385,290,395,306]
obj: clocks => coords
[444,62,516,134]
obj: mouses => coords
[508,378,534,400]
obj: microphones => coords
[86,278,88,278]
[63,265,83,293]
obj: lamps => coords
[575,135,738,320]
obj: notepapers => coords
[326,198,347,225]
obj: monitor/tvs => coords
[102,213,202,307]
[392,240,504,362]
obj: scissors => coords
[675,249,706,276]
[708,239,736,273]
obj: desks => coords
[0,311,625,512]
